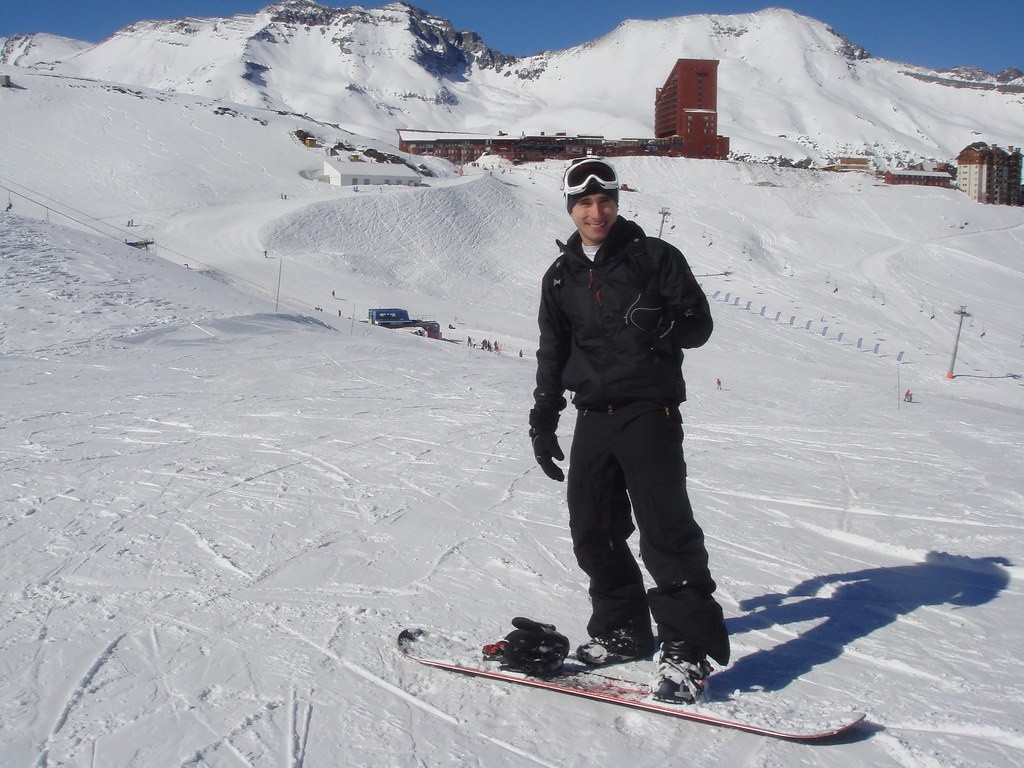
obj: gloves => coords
[623,292,664,342]
[528,408,565,482]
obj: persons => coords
[528,156,731,704]
[903,390,913,402]
[468,335,523,358]
[4,203,12,211]
[717,379,721,389]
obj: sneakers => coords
[652,640,714,704]
[576,615,655,666]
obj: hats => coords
[564,158,619,214]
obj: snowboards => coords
[396,624,869,742]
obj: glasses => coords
[563,159,620,203]
